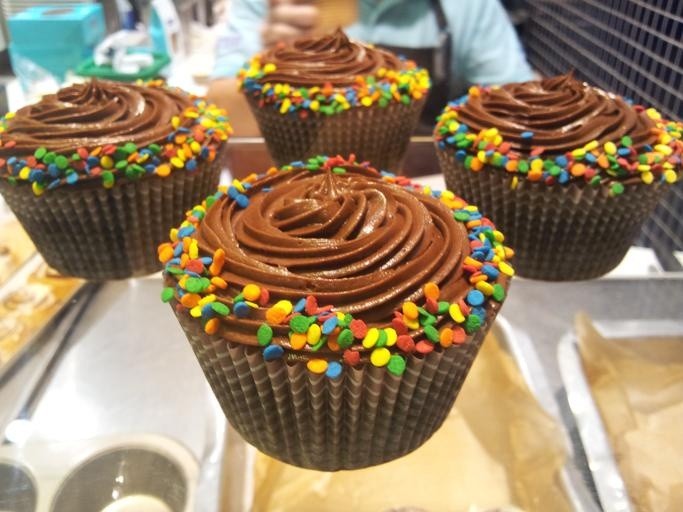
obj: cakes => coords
[236,27,433,177]
[432,73,683,282]
[0,75,234,280]
[157,154,516,472]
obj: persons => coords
[207,0,535,137]
